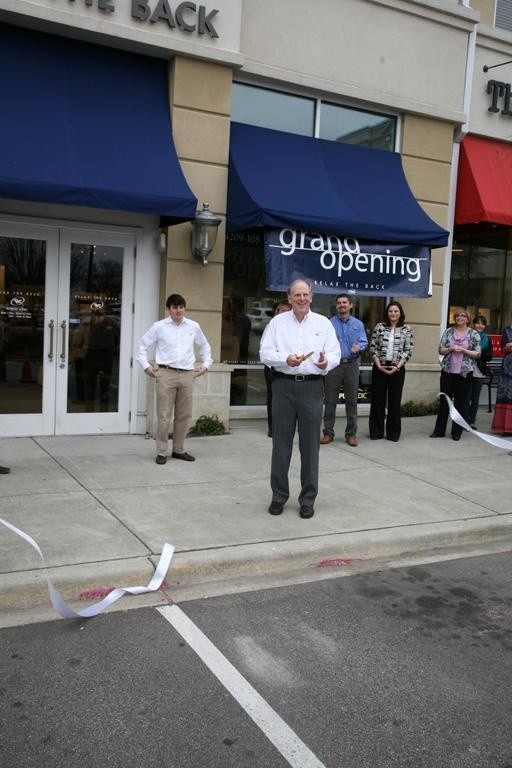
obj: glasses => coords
[455,313,469,317]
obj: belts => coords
[271,371,324,382]
[158,364,194,372]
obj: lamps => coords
[192,203,222,266]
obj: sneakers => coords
[466,425,478,432]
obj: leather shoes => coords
[370,434,383,440]
[430,433,445,438]
[345,435,357,447]
[320,434,334,444]
[171,451,195,461]
[386,436,399,442]
[156,454,168,464]
[451,432,461,440]
[340,358,357,363]
[267,431,273,438]
[299,503,314,518]
[269,500,284,515]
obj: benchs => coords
[483,360,503,413]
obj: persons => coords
[263,304,291,437]
[320,294,368,446]
[369,302,414,442]
[461,315,493,430]
[430,310,481,440]
[136,294,213,465]
[259,278,342,520]
[492,325,512,437]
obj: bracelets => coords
[395,365,400,371]
